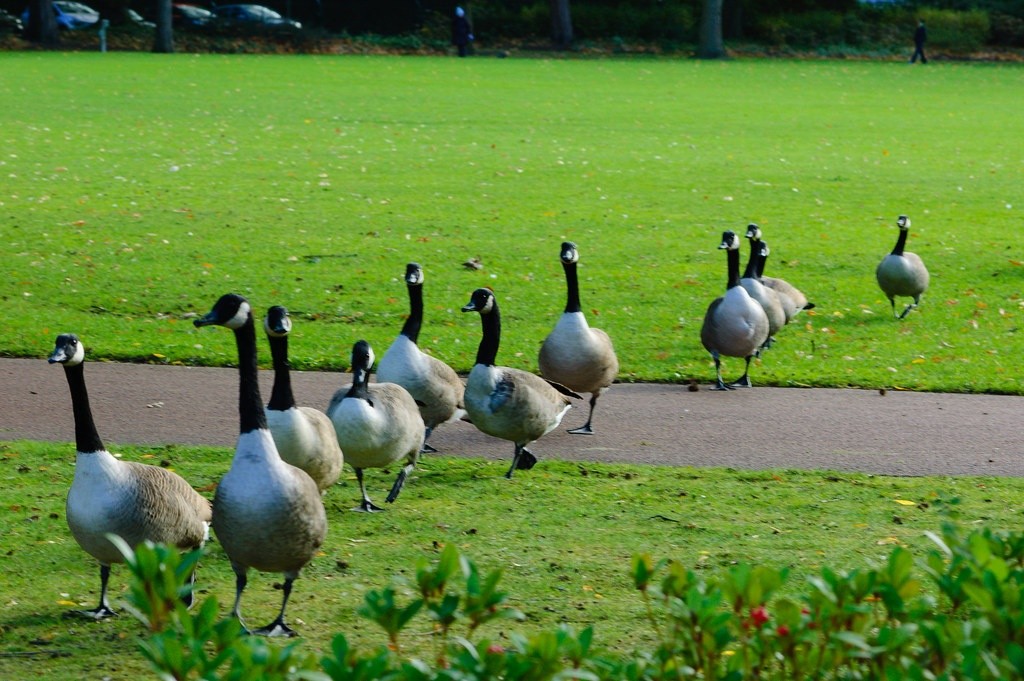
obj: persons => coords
[910,19,927,67]
[451,7,473,59]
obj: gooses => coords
[46,241,619,639]
[701,223,815,392]
[875,216,930,320]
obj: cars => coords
[173,4,235,36]
[105,7,159,31]
[21,0,101,34]
[216,3,302,36]
